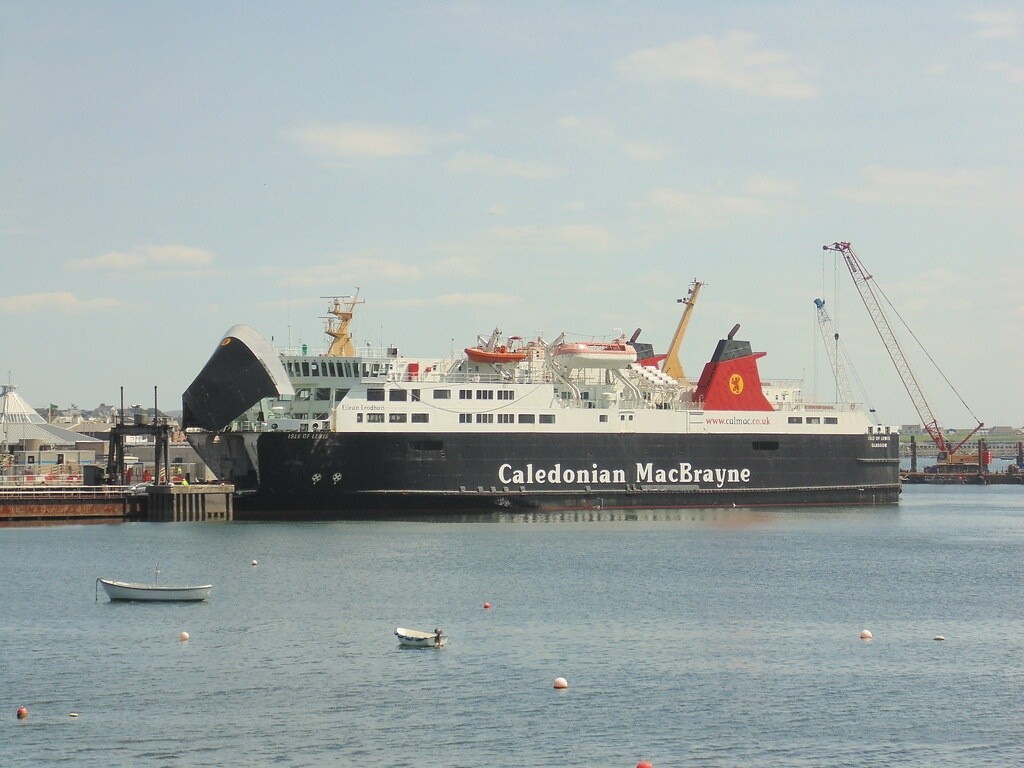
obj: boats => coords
[395,627,450,647]
[98,564,213,603]
[181,278,902,512]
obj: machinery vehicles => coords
[814,298,881,426]
[823,242,984,474]
